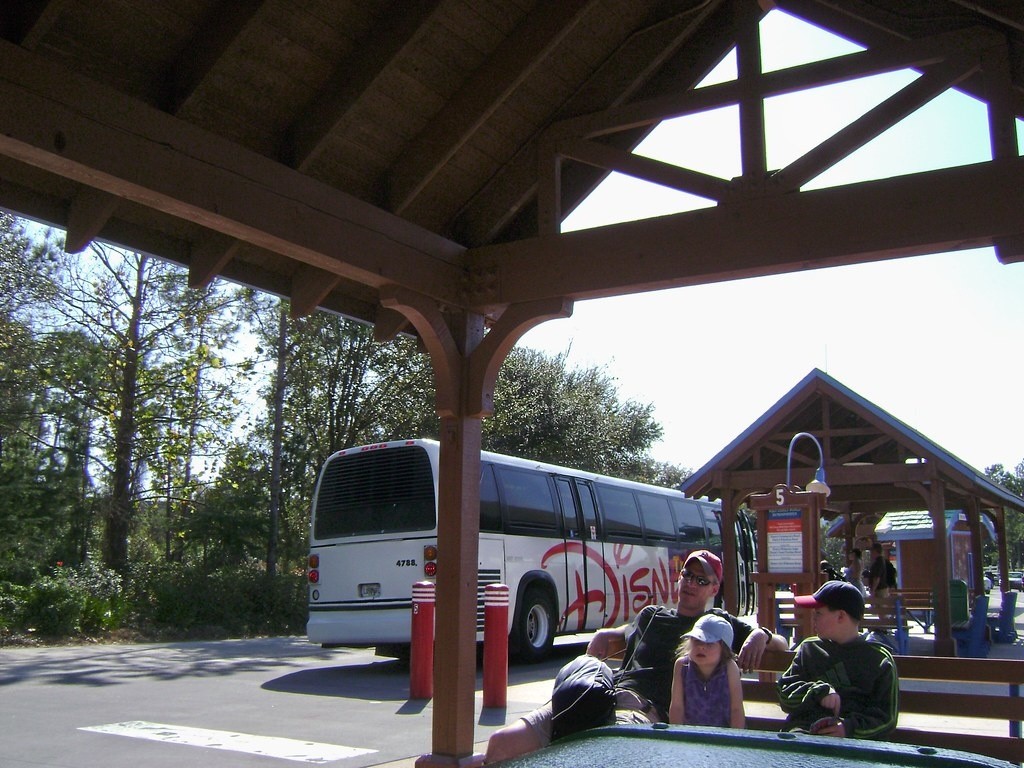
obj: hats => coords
[865,543,884,552]
[680,549,724,591]
[795,579,864,621]
[680,613,733,652]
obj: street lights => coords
[785,432,832,496]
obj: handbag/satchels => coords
[550,655,621,741]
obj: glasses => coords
[681,567,721,587]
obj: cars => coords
[998,572,1024,591]
[983,571,1000,594]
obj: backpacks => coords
[878,557,896,588]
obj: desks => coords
[476,722,1022,768]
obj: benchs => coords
[987,591,1017,642]
[952,596,992,658]
[888,588,936,634]
[606,637,1024,768]
[775,594,915,656]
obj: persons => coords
[668,614,746,729]
[820,542,891,619]
[486,548,789,766]
[776,579,900,741]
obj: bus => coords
[306,437,757,663]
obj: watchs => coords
[758,626,772,644]
